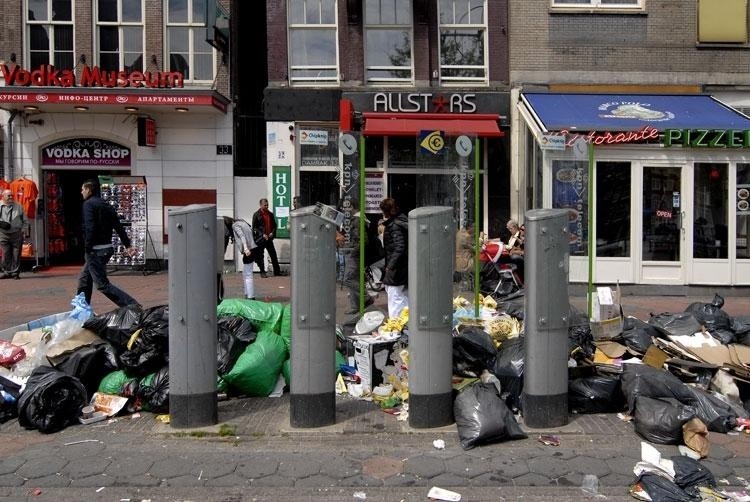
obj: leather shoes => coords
[343,306,360,315]
[261,274,268,278]
[362,296,375,307]
[275,271,289,277]
[13,274,21,280]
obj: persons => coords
[343,198,385,315]
[75,181,144,310]
[0,190,31,280]
[252,197,288,278]
[219,215,258,300]
[290,195,304,211]
[506,219,524,254]
[517,224,526,251]
[455,219,476,273]
[379,198,408,324]
